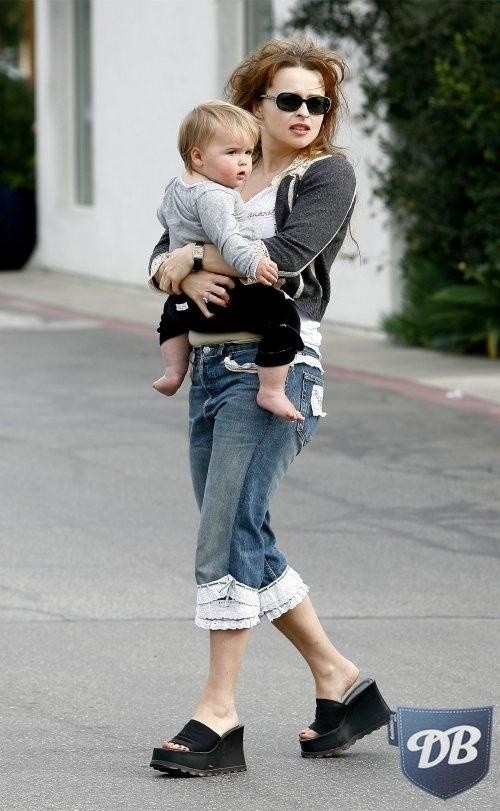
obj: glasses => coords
[262,92,332,116]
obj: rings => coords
[203,292,211,304]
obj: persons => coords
[150,98,306,426]
[143,33,393,783]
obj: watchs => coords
[192,240,205,271]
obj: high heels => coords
[299,671,394,757]
[149,718,247,778]
